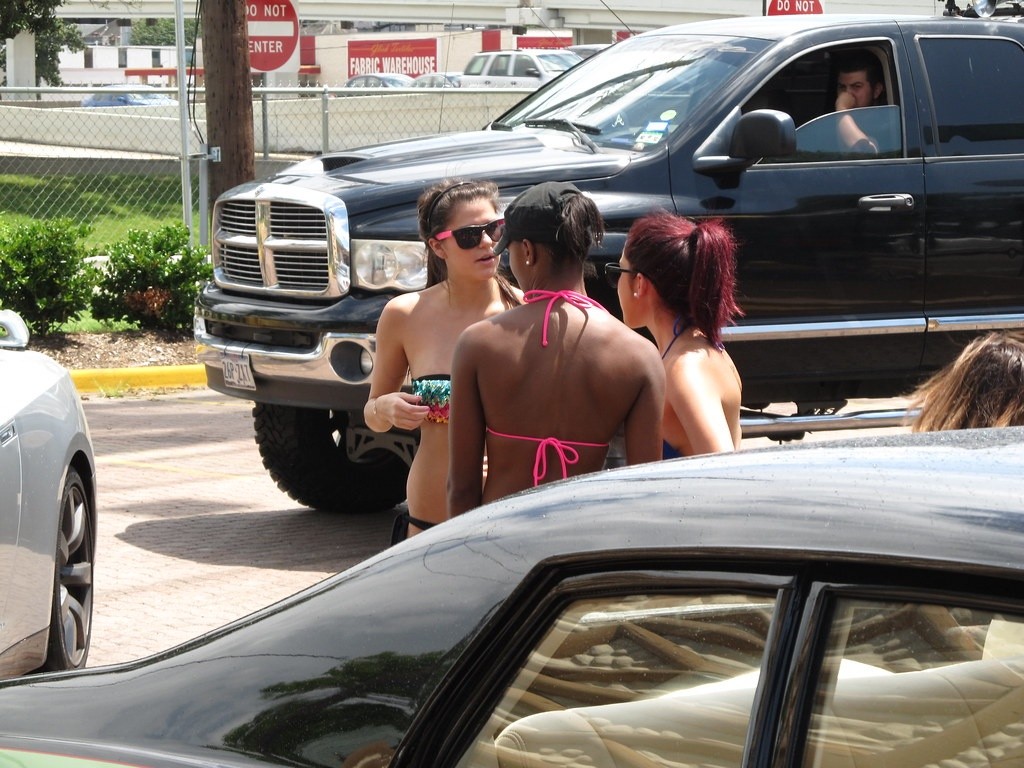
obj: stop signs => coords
[767,1,823,15]
[247,0,299,70]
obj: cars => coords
[0,308,97,679]
[342,72,460,88]
[0,427,1024,768]
[82,85,180,107]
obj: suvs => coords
[461,44,615,92]
[194,15,1024,511]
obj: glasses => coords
[605,262,656,289]
[433,219,506,250]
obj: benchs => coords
[485,658,1024,768]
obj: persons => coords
[834,63,885,157]
[445,180,666,523]
[903,328,1024,433]
[606,214,743,459]
[362,181,529,539]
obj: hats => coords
[493,181,583,256]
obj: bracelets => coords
[372,399,379,416]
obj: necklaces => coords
[662,311,725,357]
